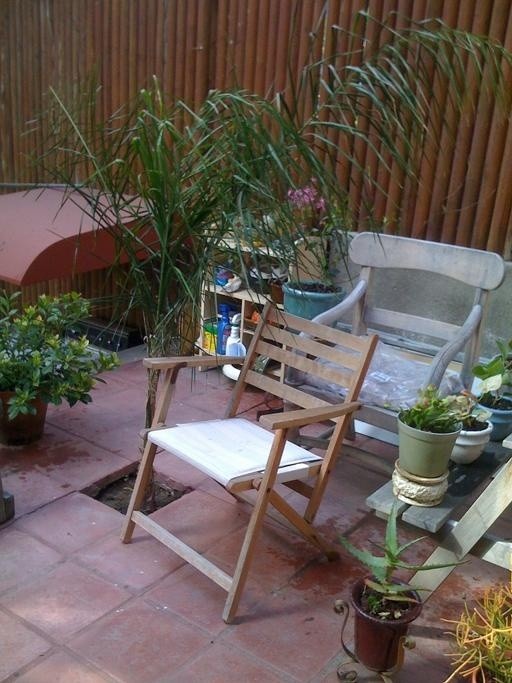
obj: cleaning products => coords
[217,303,232,354]
[225,312,242,358]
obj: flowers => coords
[288,176,327,211]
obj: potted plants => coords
[396,339,511,479]
[333,499,468,672]
[0,288,125,445]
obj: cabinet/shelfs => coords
[198,220,328,383]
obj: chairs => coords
[119,301,379,622]
[286,231,504,480]
